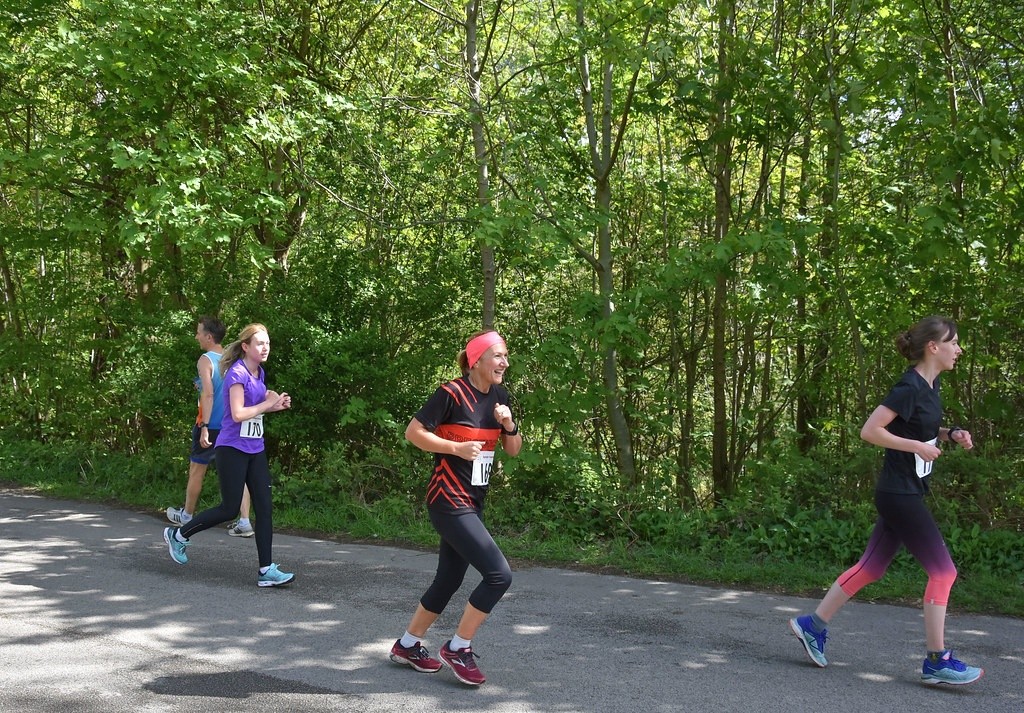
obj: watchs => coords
[200,421,209,428]
[503,421,520,436]
[947,426,961,446]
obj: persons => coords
[163,324,295,588]
[789,314,985,685]
[389,329,522,685]
[166,316,254,538]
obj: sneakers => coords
[790,615,830,668]
[163,527,189,565]
[920,649,984,686]
[257,562,295,586]
[228,523,255,537]
[166,507,193,526]
[437,640,486,685]
[389,638,443,672]
